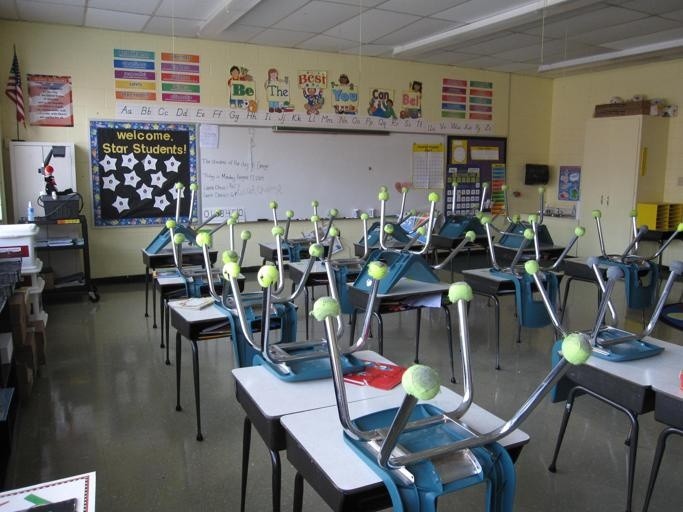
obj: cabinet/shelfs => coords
[571,114,669,281]
[19,214,100,302]
[8,141,84,286]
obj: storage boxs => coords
[0,223,49,456]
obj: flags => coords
[5,48,25,128]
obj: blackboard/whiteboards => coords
[196,124,447,225]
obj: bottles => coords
[51,191,57,200]
[543,204,576,217]
[27,201,35,222]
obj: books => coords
[344,359,406,390]
[198,320,232,339]
[35,238,85,247]
[381,300,400,306]
[57,219,80,224]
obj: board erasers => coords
[257,219,268,221]
